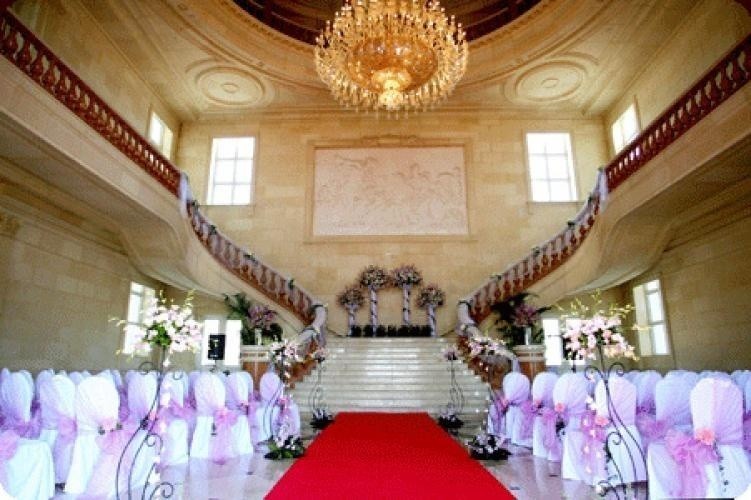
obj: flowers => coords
[264,338,300,372]
[387,262,422,287]
[331,286,365,307]
[462,332,510,361]
[551,286,650,365]
[357,265,389,289]
[308,337,331,363]
[513,299,536,325]
[440,338,461,362]
[247,302,281,332]
[104,282,206,369]
[413,280,448,310]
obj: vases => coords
[523,327,533,346]
[255,329,262,345]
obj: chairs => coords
[0,365,301,500]
[484,361,751,500]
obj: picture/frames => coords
[302,134,479,245]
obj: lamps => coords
[311,1,472,123]
